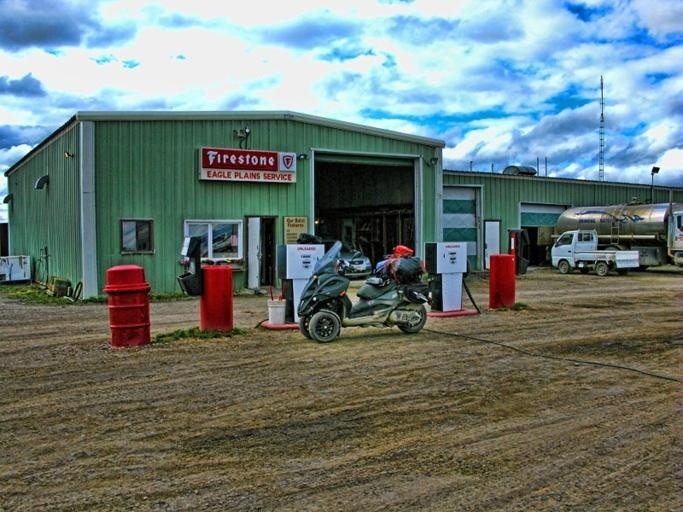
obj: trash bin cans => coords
[231,264,246,293]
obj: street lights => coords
[650,166,660,203]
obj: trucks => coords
[556,203,683,272]
[550,228,640,276]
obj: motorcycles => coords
[297,240,433,343]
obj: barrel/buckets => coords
[102,263,151,347]
[267,299,287,325]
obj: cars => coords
[335,249,372,279]
